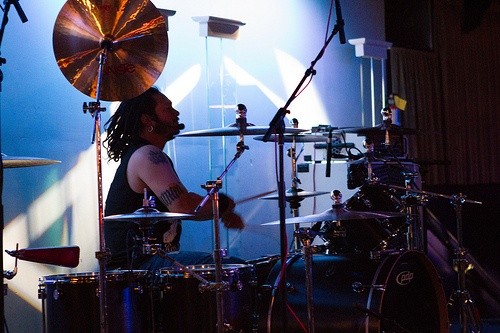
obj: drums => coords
[38,268,153,333]
[316,191,402,259]
[255,250,450,333]
[153,263,255,333]
[370,162,423,206]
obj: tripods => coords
[367,178,484,333]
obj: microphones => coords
[335,0,346,44]
[326,132,333,177]
[10,0,28,23]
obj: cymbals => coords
[173,126,311,137]
[253,135,328,143]
[102,208,196,221]
[52,0,169,103]
[262,190,330,198]
[338,123,413,135]
[1,152,63,169]
[260,206,408,226]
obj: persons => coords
[103,88,247,271]
